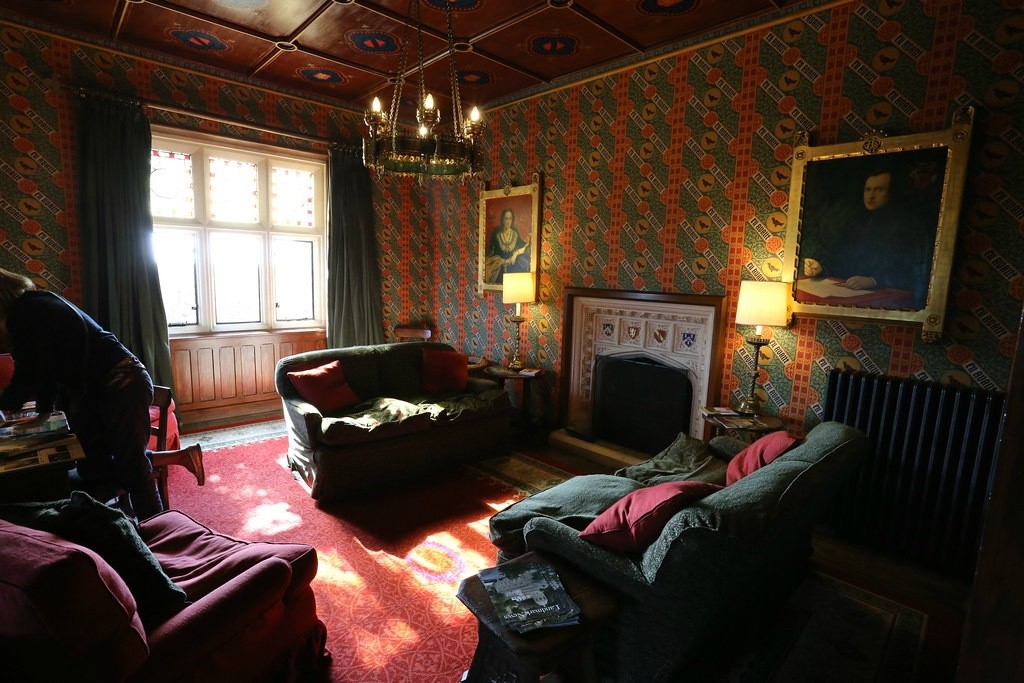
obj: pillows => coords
[0,491,192,621]
[576,480,722,552]
[726,430,804,487]
[422,349,470,393]
[286,359,359,415]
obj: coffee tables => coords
[463,549,621,683]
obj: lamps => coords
[735,280,789,416]
[362,0,485,187]
[502,272,534,370]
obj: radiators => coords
[821,367,1009,584]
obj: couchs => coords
[491,421,866,683]
[0,511,332,683]
[275,342,518,498]
[0,353,180,451]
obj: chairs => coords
[394,328,431,343]
[150,384,172,510]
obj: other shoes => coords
[186,444,206,487]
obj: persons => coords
[0,266,205,515]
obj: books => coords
[699,404,769,430]
[478,549,582,634]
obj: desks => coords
[484,364,544,436]
[702,411,783,441]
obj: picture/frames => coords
[781,105,976,344]
[477,172,540,304]
